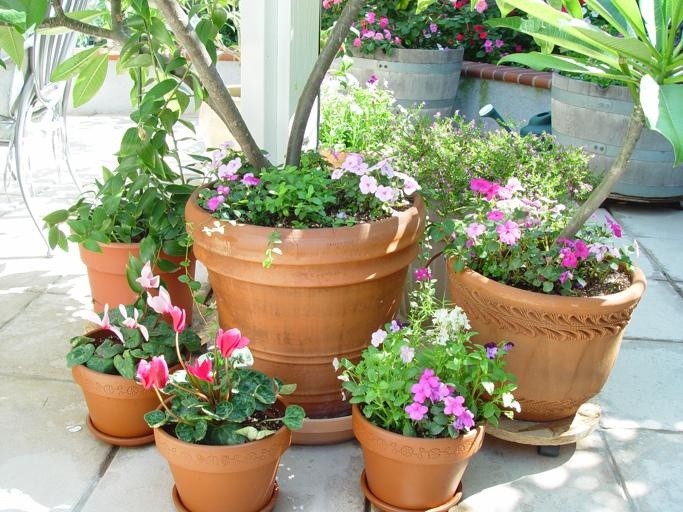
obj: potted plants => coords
[31,46,199,331]
[544,0,682,211]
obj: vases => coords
[352,404,487,512]
[154,395,292,512]
[68,331,187,441]
[335,43,466,122]
[184,175,427,416]
[446,250,650,421]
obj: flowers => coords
[317,0,524,56]
[330,264,524,438]
[69,262,175,347]
[453,172,627,287]
[137,305,258,399]
[206,152,423,218]
